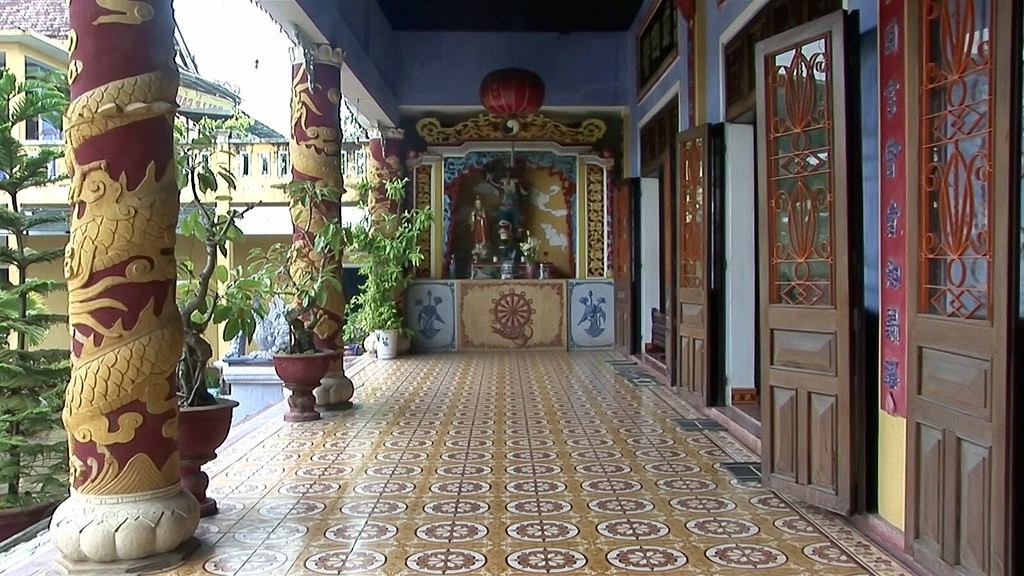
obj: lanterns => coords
[479,67,546,116]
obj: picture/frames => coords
[636,0,678,103]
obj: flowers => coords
[520,230,539,266]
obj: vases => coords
[527,263,535,280]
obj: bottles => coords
[450,255,456,279]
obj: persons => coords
[469,200,492,250]
[488,166,530,248]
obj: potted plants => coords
[236,178,357,423]
[171,120,259,517]
[339,178,436,361]
[0,71,78,539]
